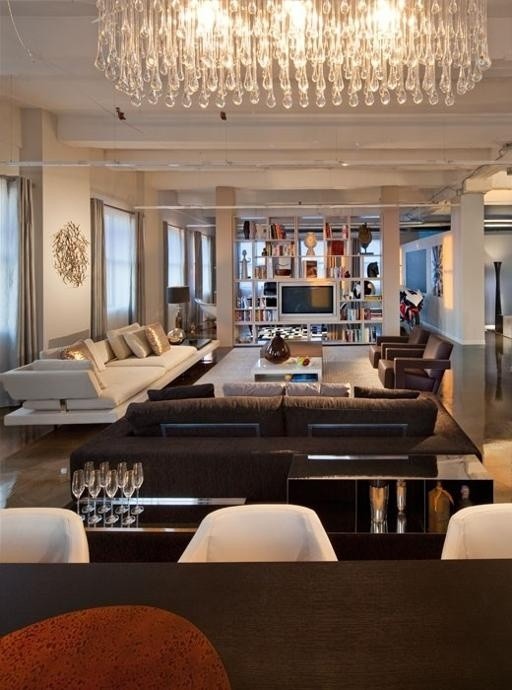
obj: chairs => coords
[378,336,453,389]
[440,503,511,560]
[0,508,90,563]
[176,504,339,563]
[369,326,429,368]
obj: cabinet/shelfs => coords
[233,214,384,345]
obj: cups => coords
[344,295,352,300]
[370,479,389,524]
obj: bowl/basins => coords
[276,269,291,276]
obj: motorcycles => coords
[399,288,425,332]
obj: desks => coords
[284,453,494,536]
[66,498,247,561]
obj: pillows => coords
[32,322,170,388]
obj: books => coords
[328,257,341,278]
[367,308,384,321]
[255,310,273,321]
[235,295,252,321]
[266,242,295,256]
[327,324,382,343]
[340,301,360,320]
[255,265,267,279]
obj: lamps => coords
[95,0,492,108]
[168,287,189,328]
[494,261,503,332]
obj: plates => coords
[167,328,186,345]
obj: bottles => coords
[396,479,407,510]
[261,241,295,256]
[236,297,252,343]
[244,221,266,240]
[330,265,350,278]
[188,324,197,346]
[304,232,317,255]
[428,482,472,522]
[255,298,272,322]
[255,266,266,279]
[241,250,248,279]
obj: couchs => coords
[71,394,482,502]
[0,340,220,435]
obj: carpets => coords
[193,346,383,397]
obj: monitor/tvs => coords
[278,283,336,317]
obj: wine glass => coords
[72,461,144,524]
